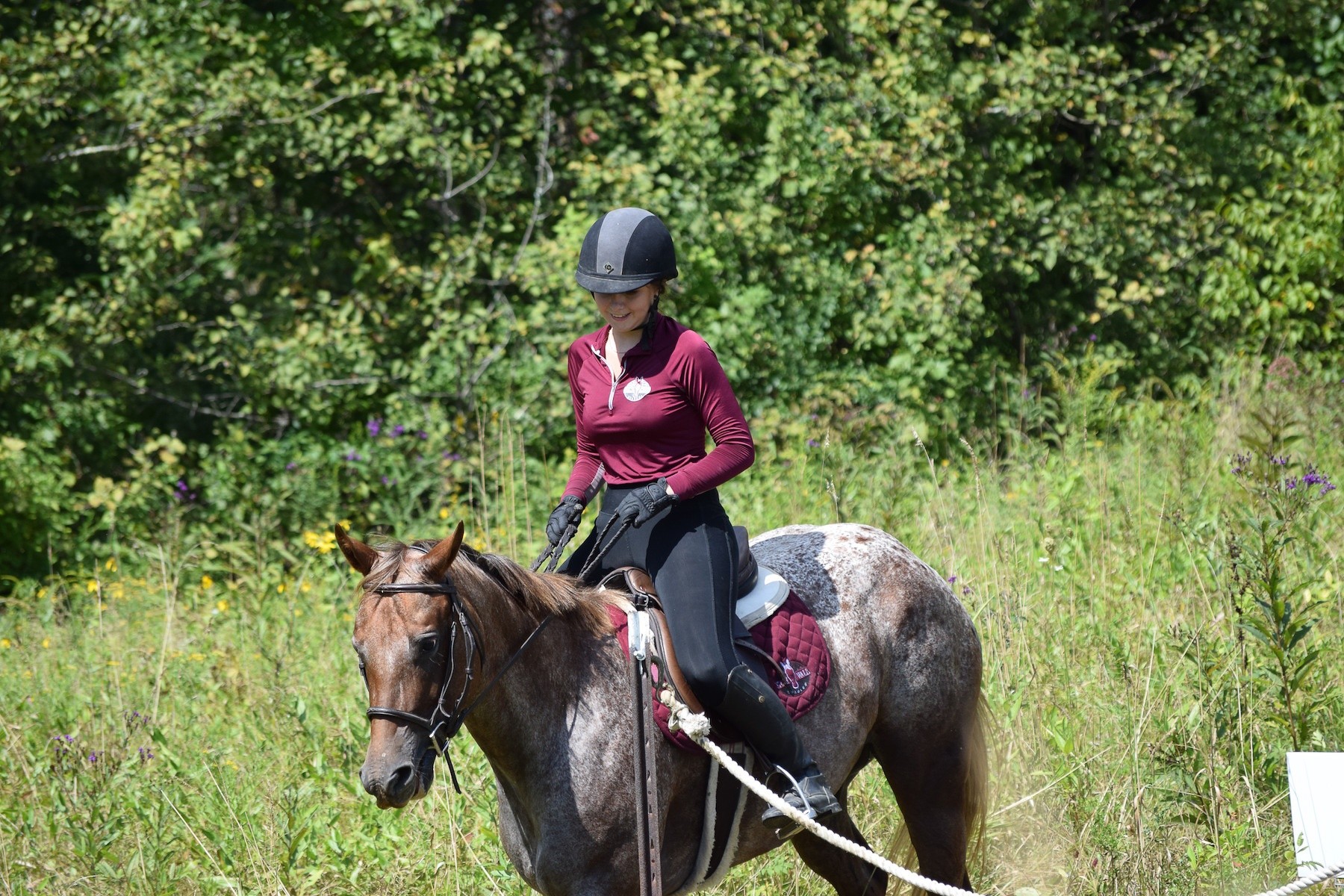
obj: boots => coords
[720,660,841,833]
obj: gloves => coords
[616,476,675,528]
[546,495,583,546]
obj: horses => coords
[334,519,994,896]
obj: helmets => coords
[575,208,677,294]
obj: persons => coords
[547,205,843,822]
